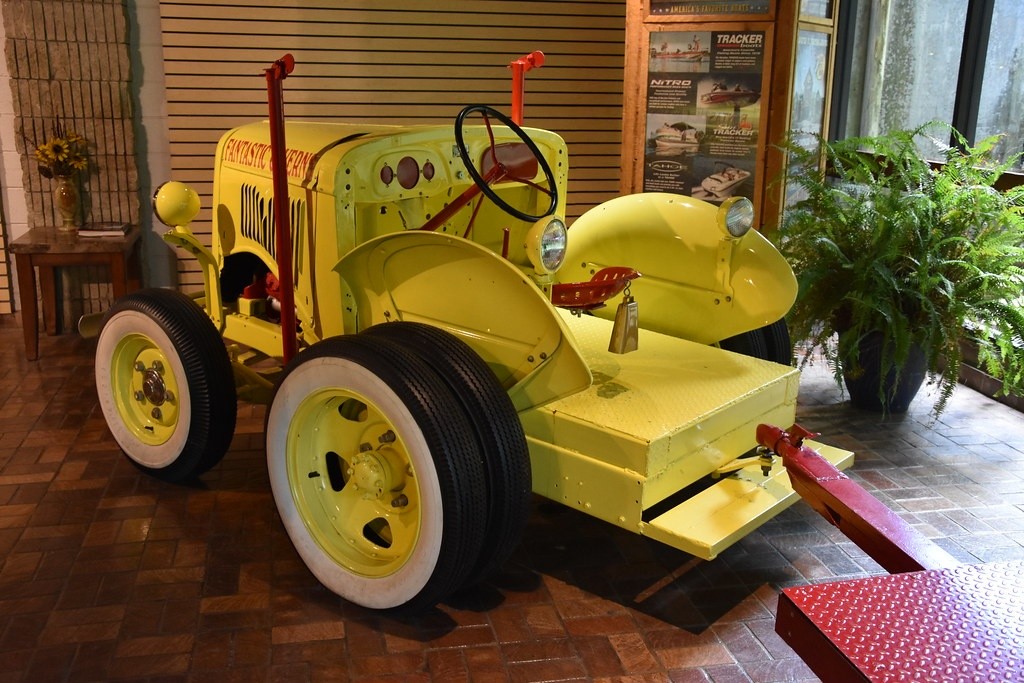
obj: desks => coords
[8,223,141,362]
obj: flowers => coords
[16,119,90,178]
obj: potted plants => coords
[761,117,1024,415]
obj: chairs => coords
[545,264,642,318]
